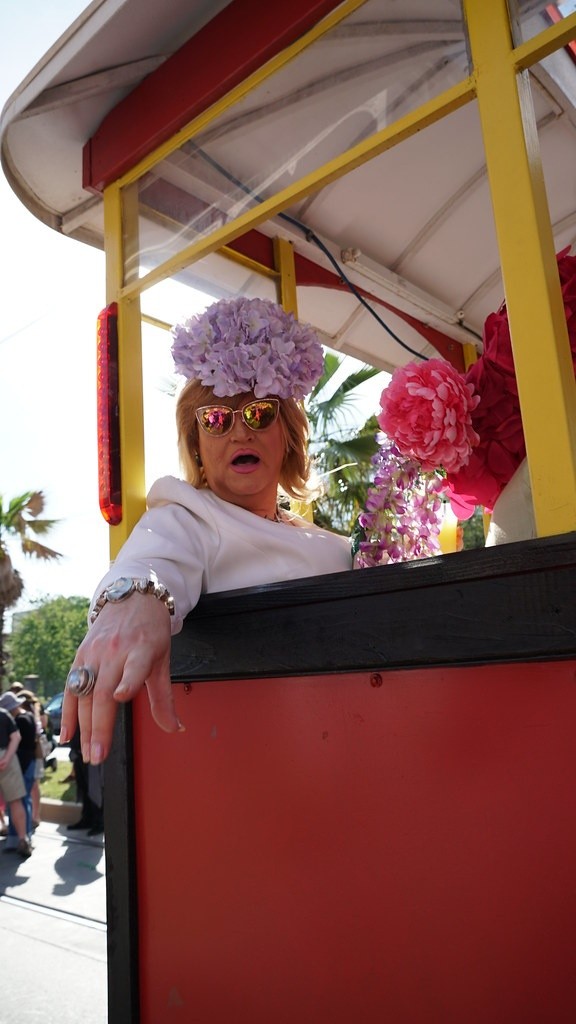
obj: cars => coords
[44,691,64,736]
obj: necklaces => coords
[265,504,285,523]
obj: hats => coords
[17,691,37,704]
[0,692,26,711]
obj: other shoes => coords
[68,819,89,830]
[16,838,32,858]
[59,775,75,784]
[50,758,57,771]
[0,827,10,836]
[31,819,39,829]
[88,824,104,836]
[4,846,16,853]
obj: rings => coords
[66,665,94,696]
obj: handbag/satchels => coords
[35,740,43,759]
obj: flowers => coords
[351,244,576,569]
[172,298,323,399]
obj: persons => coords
[0,681,58,857]
[59,297,354,765]
[63,724,106,836]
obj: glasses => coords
[195,399,280,438]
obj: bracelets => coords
[90,577,176,624]
